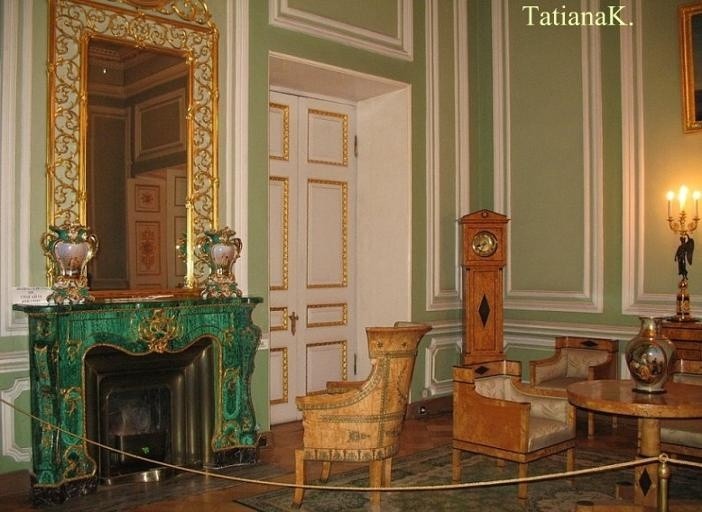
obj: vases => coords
[192,225,244,301]
[39,219,101,306]
[626,316,678,395]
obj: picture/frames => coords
[676,3,702,136]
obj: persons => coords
[674,233,694,282]
[629,353,663,375]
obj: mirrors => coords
[43,0,222,296]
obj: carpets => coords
[232,441,702,511]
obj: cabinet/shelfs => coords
[636,315,702,460]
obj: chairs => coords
[450,335,620,499]
[294,322,434,507]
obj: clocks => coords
[456,209,512,366]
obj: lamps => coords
[666,185,701,325]
